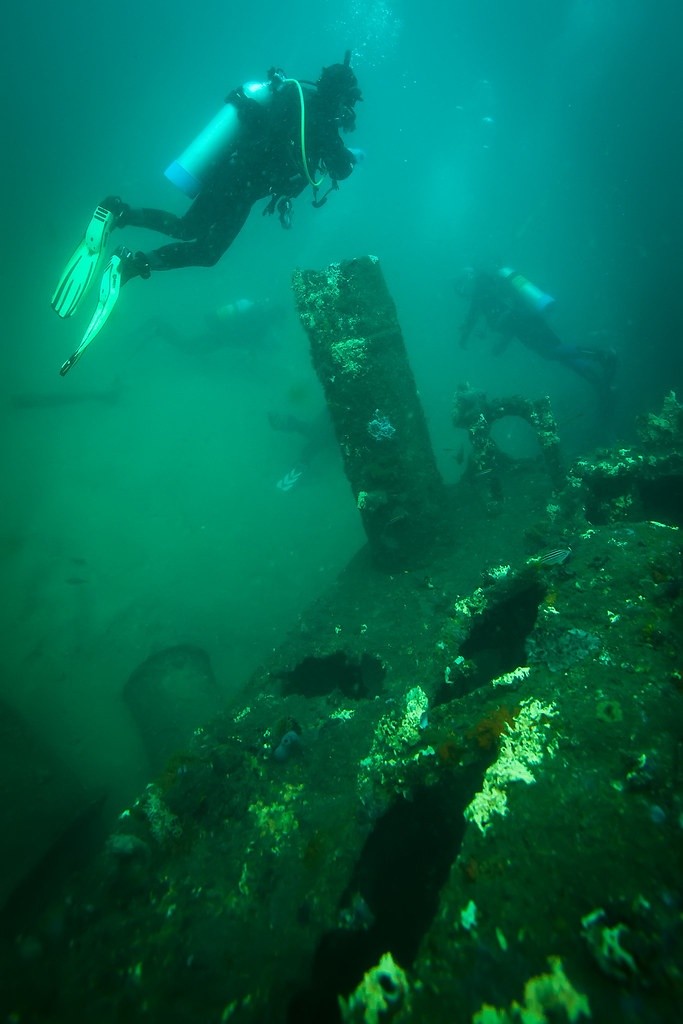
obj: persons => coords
[461,258,620,399]
[85,62,365,303]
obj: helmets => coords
[318,63,359,122]
